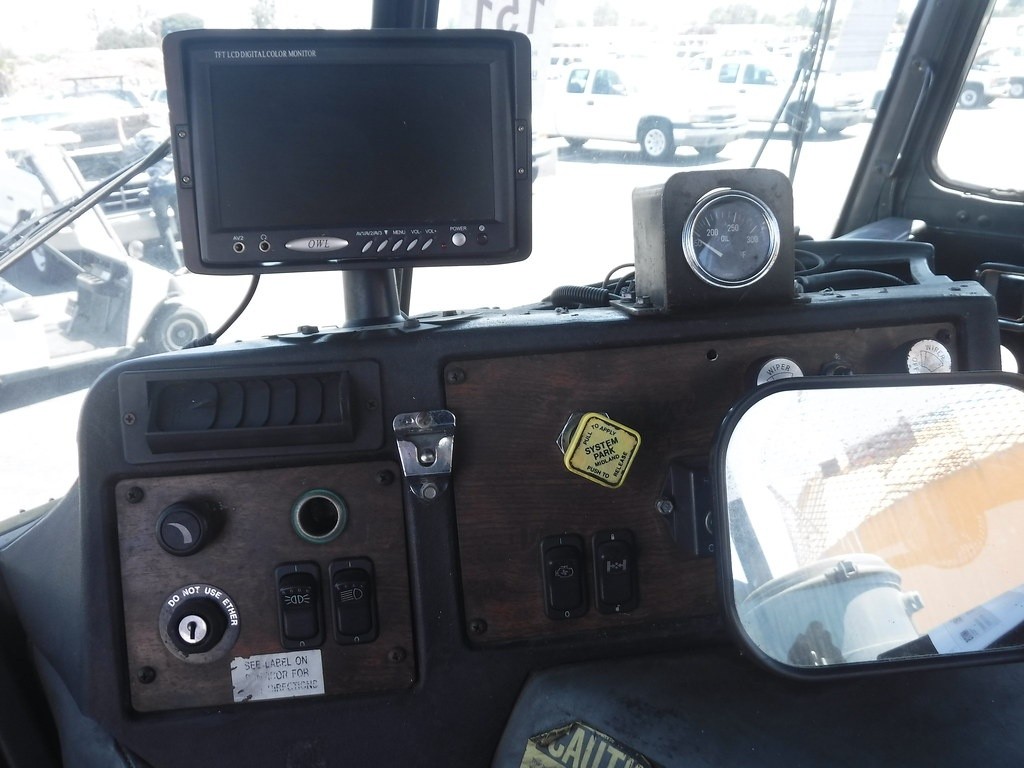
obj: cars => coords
[0,19,1024,290]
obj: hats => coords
[147,102,169,126]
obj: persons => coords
[0,58,11,99]
[114,98,188,276]
